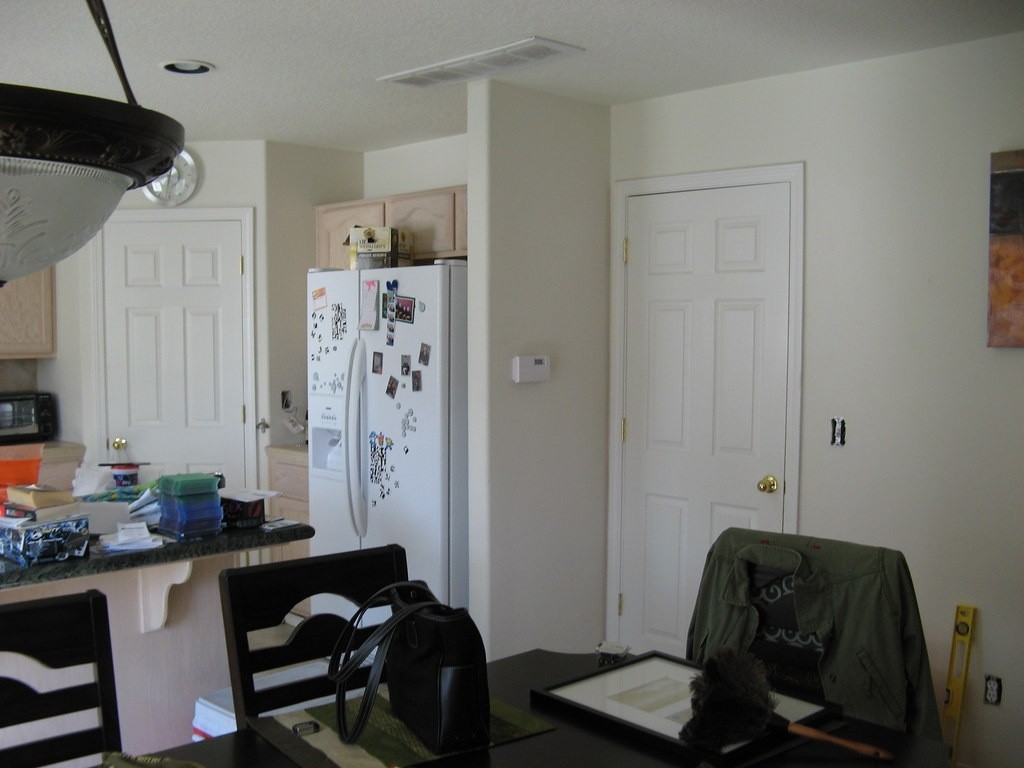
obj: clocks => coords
[142,151,197,207]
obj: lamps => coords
[0,0,186,288]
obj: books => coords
[0,441,86,526]
[99,533,166,552]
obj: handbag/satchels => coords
[328,581,489,756]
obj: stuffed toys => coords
[679,646,776,752]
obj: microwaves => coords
[1,392,59,443]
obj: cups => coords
[111,465,139,485]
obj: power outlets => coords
[982,673,1002,706]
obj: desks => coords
[94,645,951,768]
[0,514,316,768]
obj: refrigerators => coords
[306,259,468,654]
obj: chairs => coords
[686,527,944,743]
[0,589,122,768]
[218,544,408,732]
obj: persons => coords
[374,355,380,374]
[386,382,395,395]
[388,303,394,322]
[396,301,411,321]
[386,323,394,346]
[402,355,410,375]
[413,372,421,391]
[332,303,346,340]
[419,345,429,365]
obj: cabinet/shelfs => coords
[306,183,468,668]
